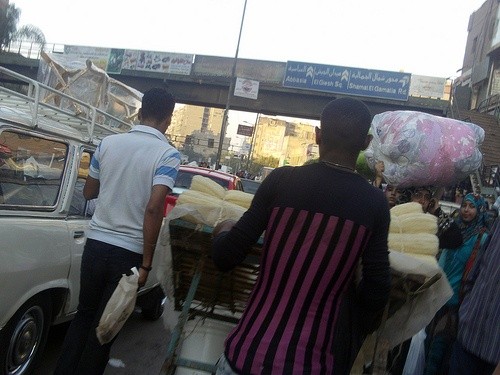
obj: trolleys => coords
[162,219,442,374]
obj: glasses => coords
[414,193,432,199]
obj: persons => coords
[387,186,462,374]
[448,216,500,375]
[422,192,490,375]
[54,89,181,375]
[212,98,391,375]
[371,161,402,209]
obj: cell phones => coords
[426,202,432,211]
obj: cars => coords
[165,165,262,232]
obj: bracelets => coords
[140,264,152,272]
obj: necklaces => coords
[320,160,355,174]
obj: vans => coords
[0,66,165,375]
